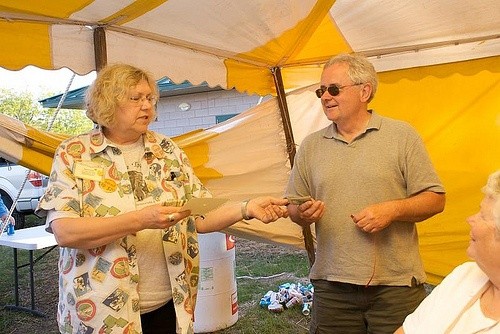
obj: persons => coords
[0,193,15,234]
[283,54,445,334]
[35,64,289,334]
[393,171,500,334]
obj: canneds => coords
[258,282,314,316]
[7,221,14,235]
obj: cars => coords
[0,155,52,230]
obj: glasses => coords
[124,96,158,108]
[314,83,362,98]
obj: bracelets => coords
[242,199,254,220]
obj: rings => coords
[169,214,174,222]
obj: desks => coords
[0,226,58,315]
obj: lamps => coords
[179,103,191,111]
